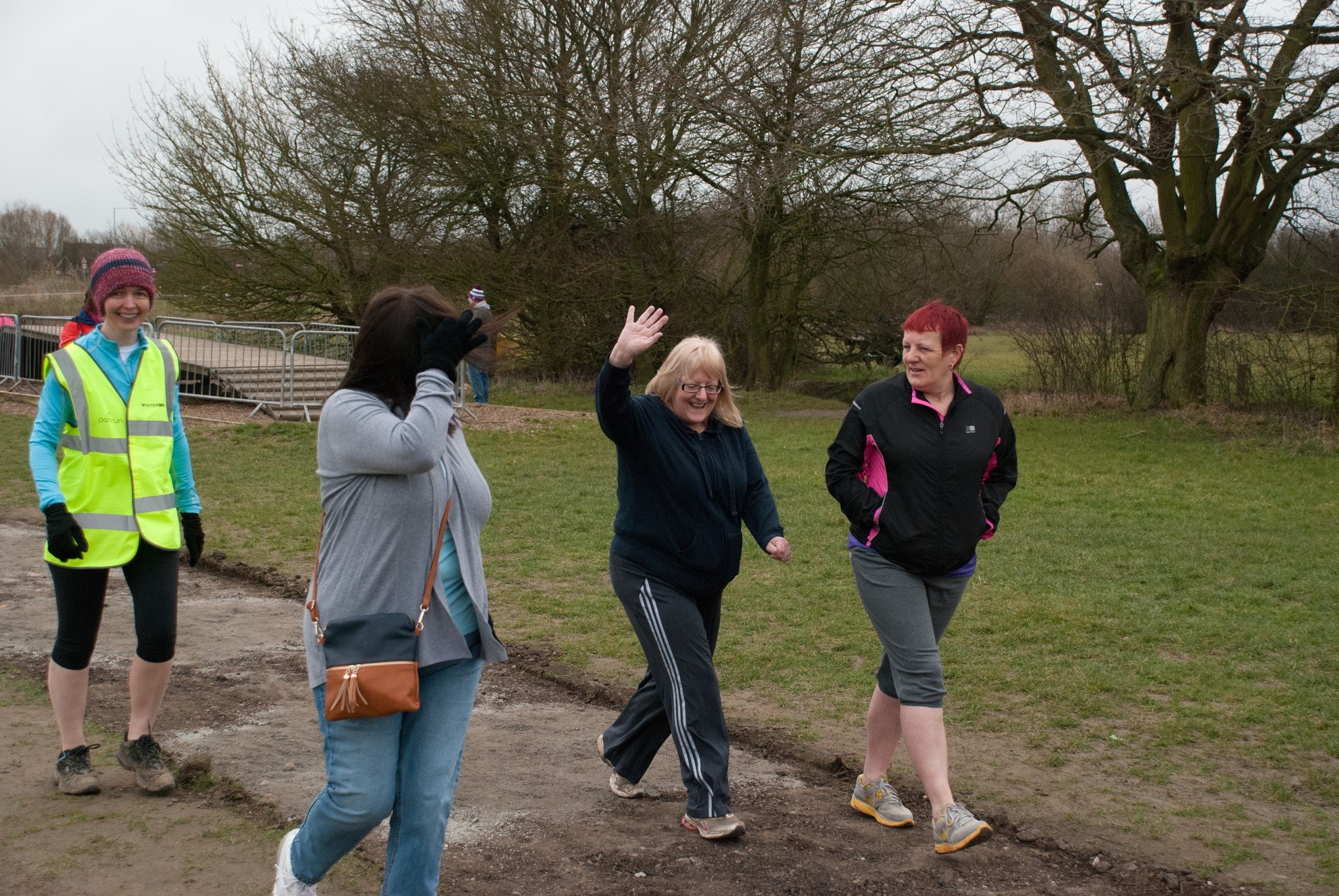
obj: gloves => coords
[181,511,207,567]
[420,310,489,372]
[45,501,89,561]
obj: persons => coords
[59,288,105,348]
[824,304,1019,855]
[595,306,793,839]
[463,286,493,404]
[272,283,508,896]
[28,249,206,796]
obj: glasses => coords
[677,379,724,394]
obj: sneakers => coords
[850,773,914,828]
[682,810,745,840]
[56,742,102,796]
[597,733,645,798]
[932,802,993,854]
[271,827,320,896]
[115,728,176,792]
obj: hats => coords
[89,247,157,316]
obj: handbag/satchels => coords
[319,612,420,721]
